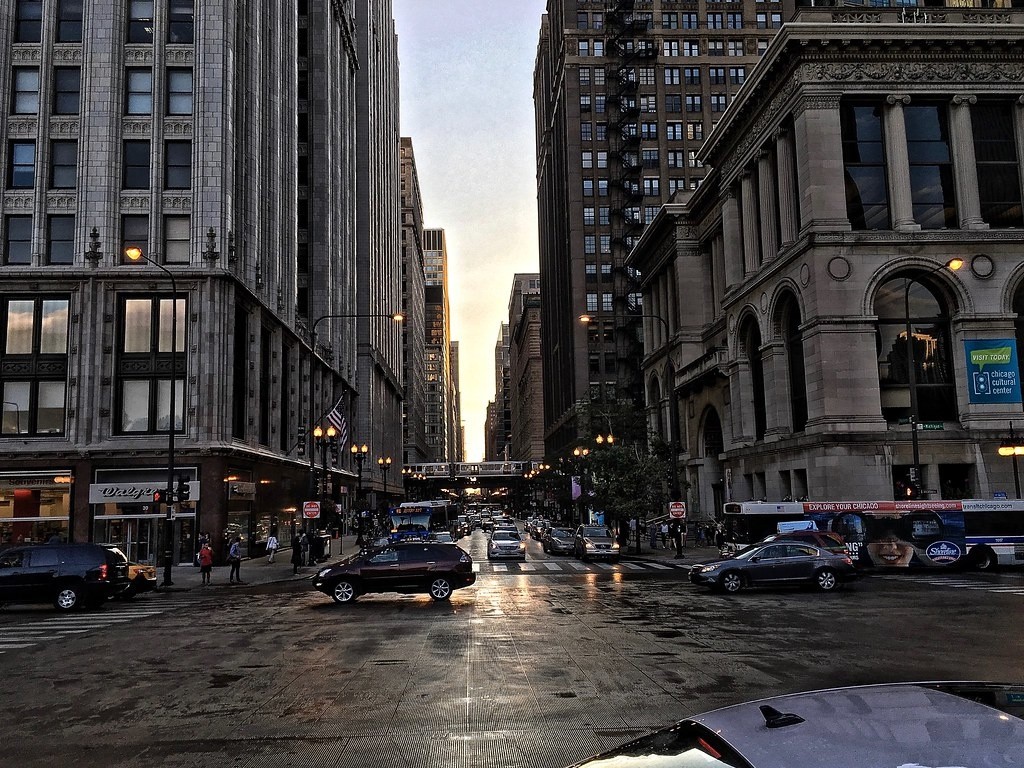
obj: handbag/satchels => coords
[226,545,237,564]
[272,543,277,549]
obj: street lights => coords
[523,434,614,519]
[350,443,427,543]
[313,425,336,531]
[905,259,963,498]
[126,248,176,584]
[579,314,686,559]
[306,315,404,564]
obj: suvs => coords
[312,539,476,604]
[762,531,855,568]
[0,541,131,615]
[575,523,620,562]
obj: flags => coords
[325,394,348,451]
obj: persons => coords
[378,552,398,562]
[266,533,279,563]
[627,517,727,551]
[351,517,391,546]
[196,532,243,586]
[291,530,325,575]
[49,532,62,545]
[770,547,780,559]
[865,513,914,568]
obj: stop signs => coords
[303,501,320,519]
[669,501,686,518]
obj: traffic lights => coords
[153,488,166,503]
[177,475,190,501]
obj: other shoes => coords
[236,580,243,582]
[230,580,234,582]
[268,561,272,564]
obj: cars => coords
[563,682,1023,768]
[524,516,577,555]
[687,540,856,593]
[486,530,526,560]
[457,509,522,537]
[125,561,156,597]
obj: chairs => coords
[764,545,787,558]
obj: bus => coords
[387,499,459,540]
[721,501,1024,570]
[468,504,502,510]
